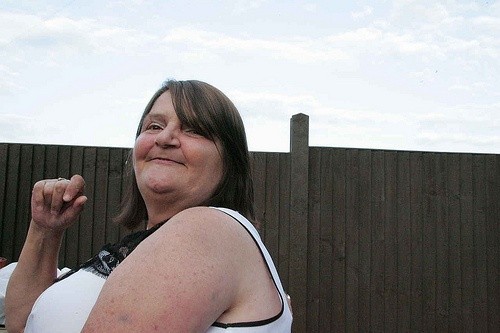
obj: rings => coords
[58,178,64,180]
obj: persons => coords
[0,80,292,333]
